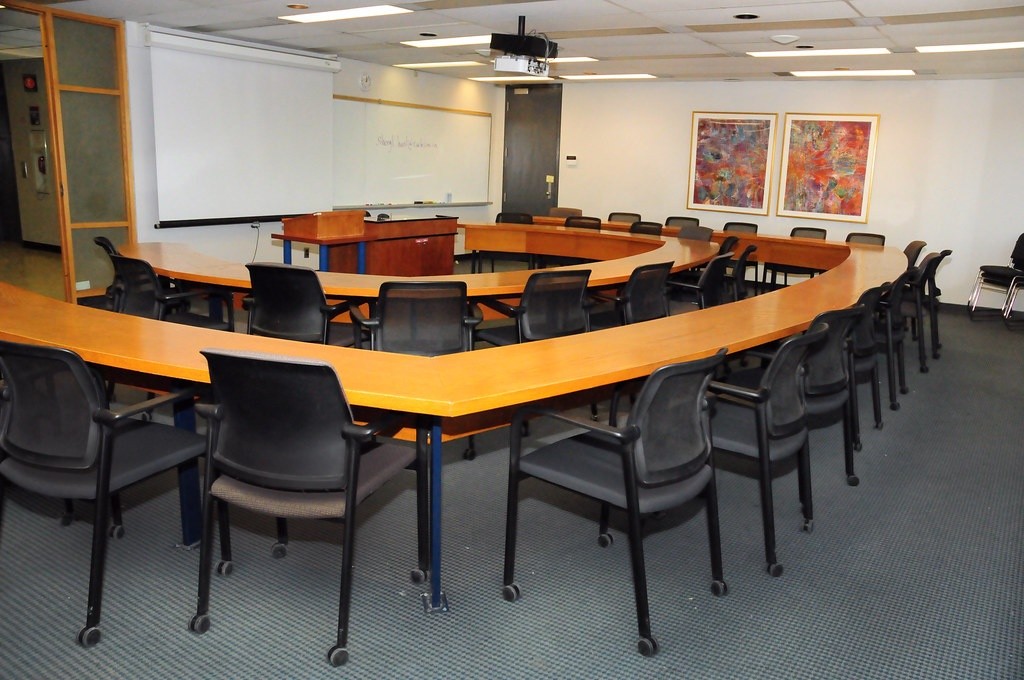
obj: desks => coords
[0,209,910,616]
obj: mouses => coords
[378,214,389,218]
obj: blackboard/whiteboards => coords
[332,95,492,210]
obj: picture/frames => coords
[686,110,779,217]
[776,112,881,224]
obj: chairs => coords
[189,347,431,667]
[495,213,534,225]
[608,212,641,223]
[679,225,714,272]
[664,216,700,228]
[548,207,582,217]
[93,236,235,332]
[964,231,1024,332]
[723,222,759,234]
[762,227,828,292]
[349,280,484,357]
[722,244,758,306]
[666,251,736,309]
[501,346,729,658]
[876,266,920,412]
[473,269,592,348]
[679,235,739,283]
[903,240,929,341]
[846,281,892,452]
[703,323,830,579]
[0,341,207,650]
[629,221,663,235]
[923,249,953,360]
[242,261,381,351]
[743,302,868,521]
[565,216,601,230]
[587,260,675,327]
[899,252,942,374]
[845,233,886,246]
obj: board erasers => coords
[423,200,433,205]
[414,201,424,204]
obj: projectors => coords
[493,57,549,77]
